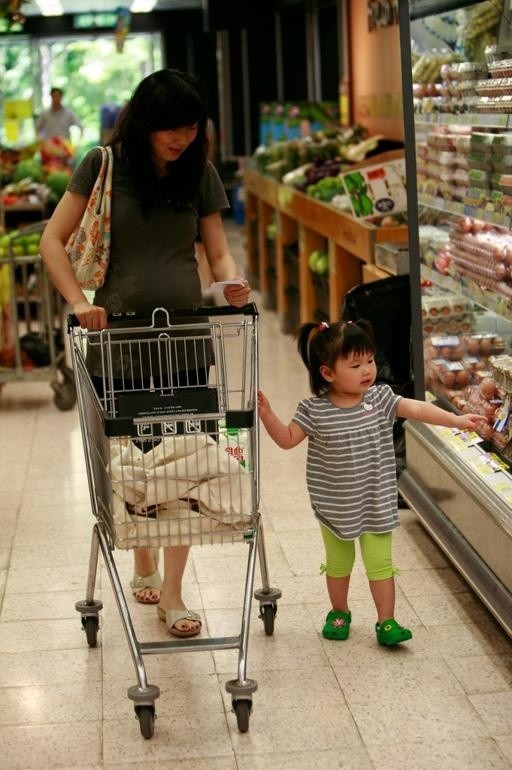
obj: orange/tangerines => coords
[0,230,40,259]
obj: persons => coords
[195,116,217,290]
[35,88,85,142]
[259,319,489,647]
[38,68,250,637]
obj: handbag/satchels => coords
[62,144,116,293]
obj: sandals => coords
[322,608,352,642]
[375,617,413,647]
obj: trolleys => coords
[0,219,80,414]
[62,301,281,742]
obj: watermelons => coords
[13,158,43,182]
[48,173,72,198]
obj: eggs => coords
[413,58,512,437]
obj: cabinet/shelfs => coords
[241,1,511,639]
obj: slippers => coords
[157,602,202,638]
[129,548,163,605]
